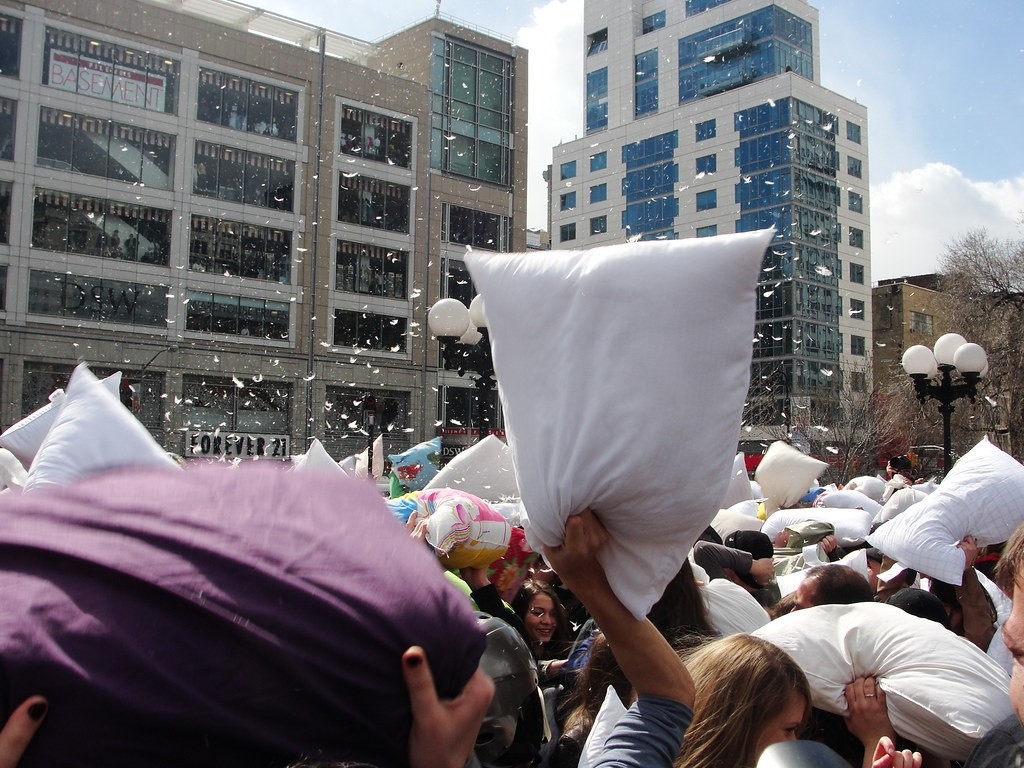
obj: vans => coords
[909,444,961,473]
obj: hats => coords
[725,529,773,590]
[866,548,884,563]
[885,588,952,631]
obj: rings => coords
[864,693,876,697]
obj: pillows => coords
[283,435,1024,763]
[0,359,180,498]
[462,229,777,622]
[0,455,487,768]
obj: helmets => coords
[474,611,539,761]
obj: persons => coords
[405,454,1024,768]
[0,644,495,768]
[111,230,140,260]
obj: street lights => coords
[428,293,498,440]
[902,332,989,479]
